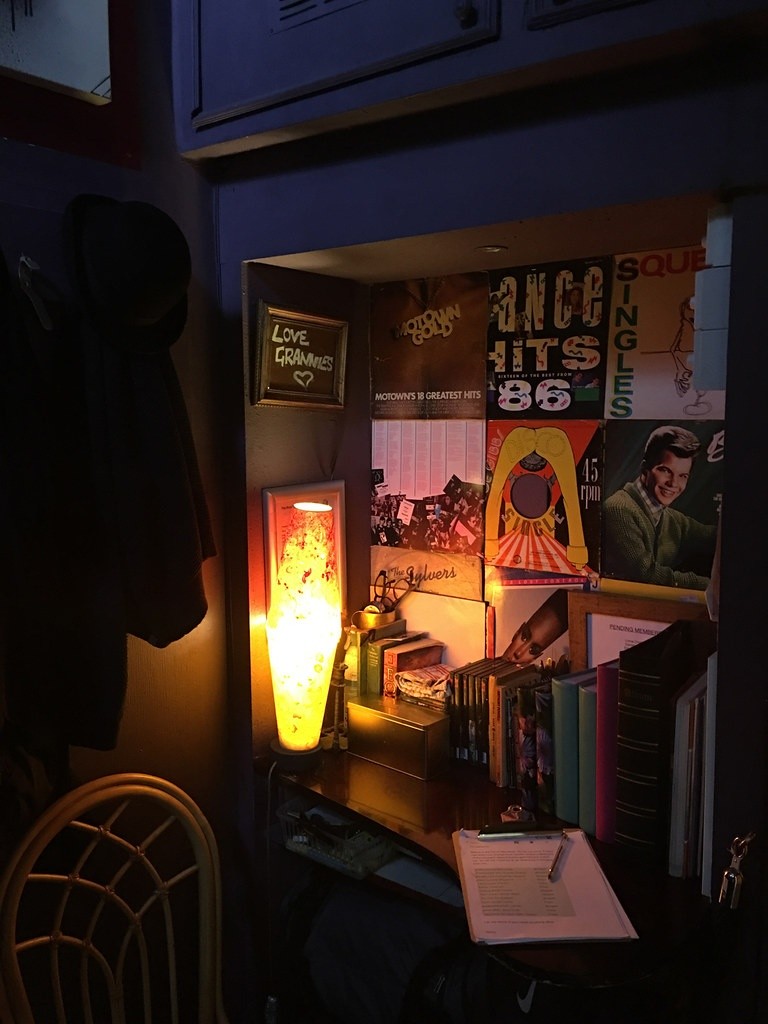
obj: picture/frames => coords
[252,298,349,412]
[260,477,348,620]
[568,590,710,674]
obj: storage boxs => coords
[346,694,450,782]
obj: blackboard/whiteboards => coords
[250,297,349,415]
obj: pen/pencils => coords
[547,834,567,879]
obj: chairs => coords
[0,772,226,1024]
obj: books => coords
[447,619,716,901]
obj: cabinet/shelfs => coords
[215,82,767,1024]
[172,0,767,162]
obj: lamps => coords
[266,608,343,775]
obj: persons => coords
[599,425,716,592]
[371,486,483,557]
[493,588,568,665]
